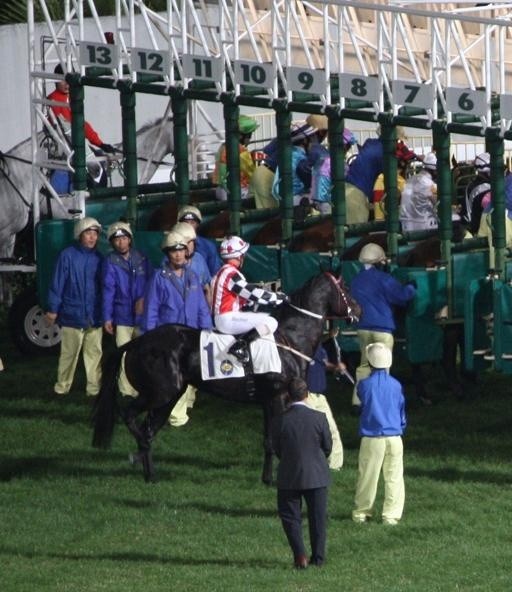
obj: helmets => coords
[475,153,491,171]
[238,114,259,132]
[73,206,250,259]
[424,153,454,170]
[359,243,385,263]
[307,115,329,131]
[376,124,405,140]
[365,342,392,369]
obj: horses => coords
[0,118,510,405]
[88,260,362,488]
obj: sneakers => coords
[295,557,307,568]
[310,557,322,565]
[84,394,101,402]
[56,394,73,407]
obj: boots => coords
[228,329,258,362]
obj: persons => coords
[46,63,117,189]
[209,115,512,272]
[210,235,290,359]
[159,222,213,289]
[351,343,406,524]
[44,218,103,398]
[271,376,332,568]
[142,233,214,429]
[346,242,417,412]
[176,205,222,272]
[102,222,152,399]
[304,342,345,473]
